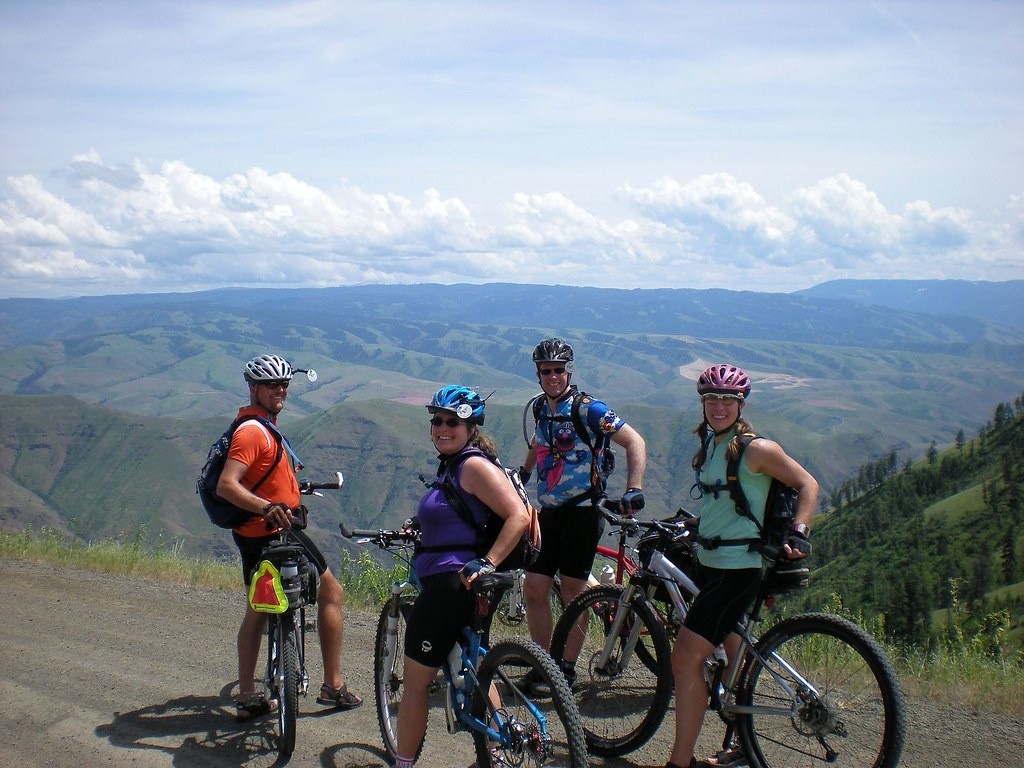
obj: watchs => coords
[792,524,810,539]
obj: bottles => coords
[448,642,465,688]
[280,561,298,578]
[599,565,615,583]
[713,641,728,666]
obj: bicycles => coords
[338,523,591,768]
[236,471,345,755]
[488,497,700,686]
[548,498,906,768]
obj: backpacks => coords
[694,432,800,543]
[533,393,616,479]
[443,448,542,570]
[195,414,284,530]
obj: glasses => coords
[703,393,740,405]
[265,381,289,389]
[429,416,459,428]
[539,366,566,375]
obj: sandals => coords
[235,695,280,724]
[316,683,363,708]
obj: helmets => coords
[531,337,575,363]
[242,354,293,386]
[425,384,487,426]
[696,363,752,404]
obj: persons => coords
[215,354,365,725]
[662,364,819,768]
[512,338,646,697]
[393,384,532,768]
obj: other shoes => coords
[700,742,748,767]
[664,755,696,768]
[512,667,579,698]
[466,748,507,768]
